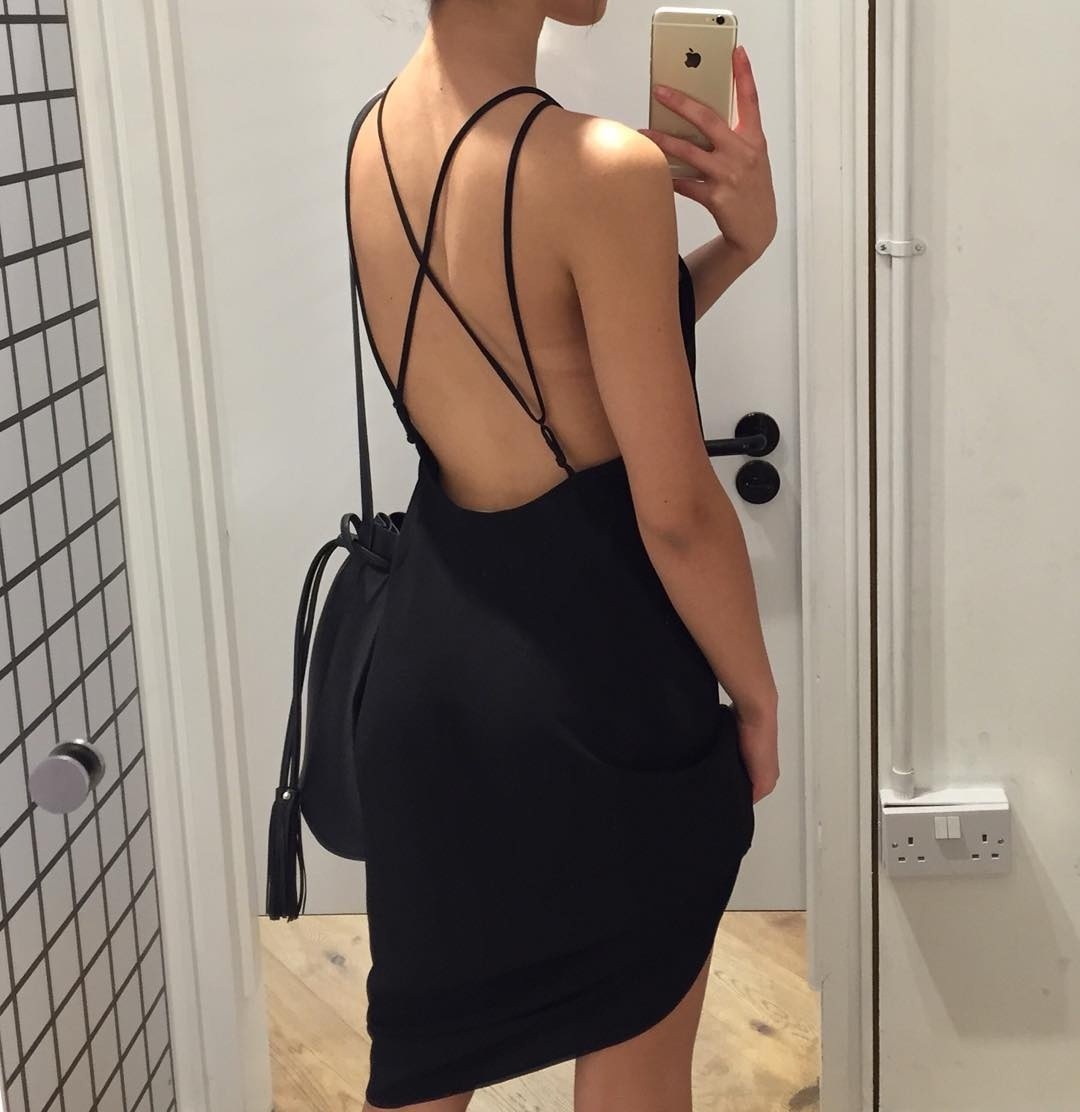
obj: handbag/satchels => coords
[256,509,409,926]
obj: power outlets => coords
[880,783,1011,880]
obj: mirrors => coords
[68,0,876,1112]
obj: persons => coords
[333,2,784,1112]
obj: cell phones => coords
[650,8,737,179]
